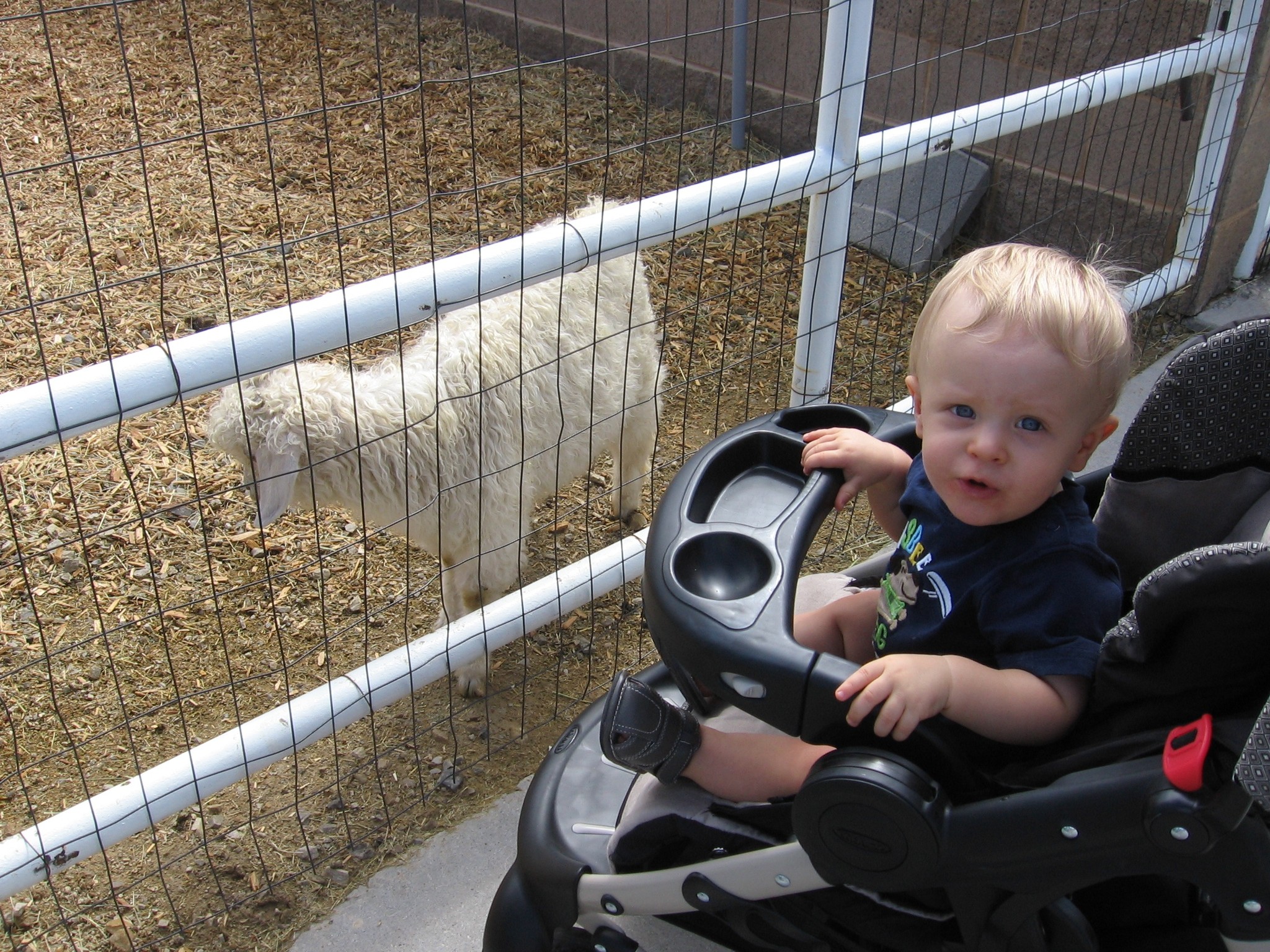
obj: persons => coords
[598,241,1133,800]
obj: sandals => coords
[599,669,702,786]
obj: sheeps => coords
[201,194,669,701]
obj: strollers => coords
[479,317,1269,952]
